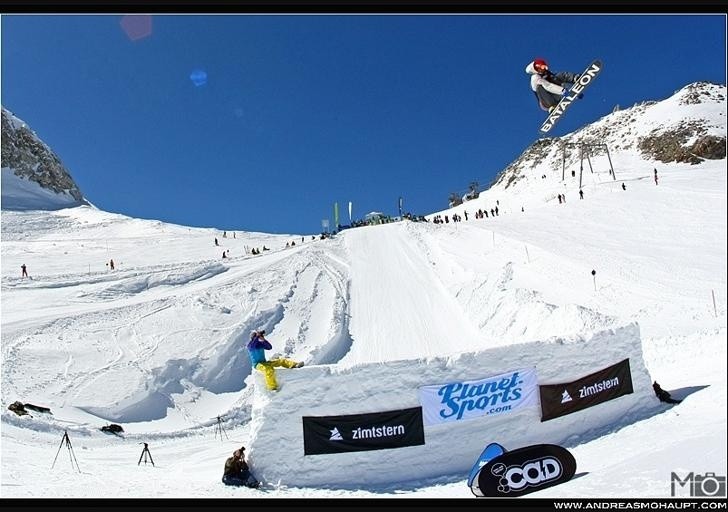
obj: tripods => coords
[138,442,154,466]
[215,416,229,442]
[51,430,80,473]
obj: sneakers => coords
[245,482,260,488]
[295,362,304,367]
[272,388,278,393]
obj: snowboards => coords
[538,58,603,135]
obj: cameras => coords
[258,330,265,335]
[239,446,245,454]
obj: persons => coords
[110,259,114,270]
[654,175,658,185]
[561,193,565,203]
[579,189,584,199]
[215,230,332,260]
[245,329,304,394]
[332,197,502,235]
[654,168,656,176]
[221,448,260,489]
[621,183,626,190]
[21,264,28,278]
[524,58,585,115]
[556,194,562,204]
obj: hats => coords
[250,330,258,340]
